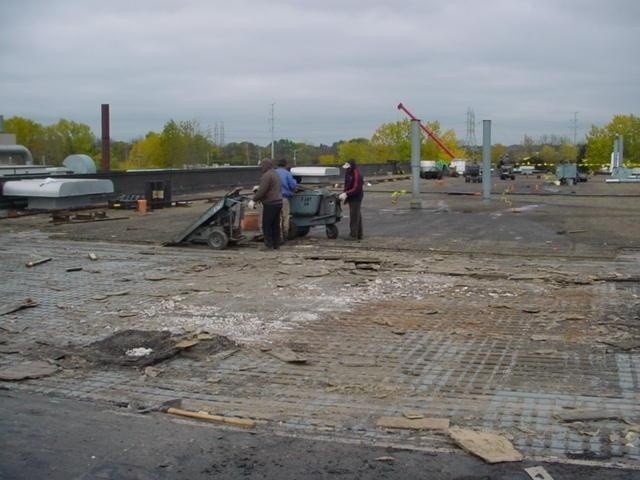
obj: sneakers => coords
[356,236,364,239]
[344,236,358,241]
[259,244,273,251]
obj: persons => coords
[337,158,364,242]
[247,157,284,252]
[274,158,297,246]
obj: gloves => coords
[247,200,256,209]
[339,192,347,201]
[253,185,260,192]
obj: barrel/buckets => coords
[243,210,261,230]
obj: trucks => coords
[556,164,589,185]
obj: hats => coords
[259,157,271,166]
[342,160,355,168]
[278,158,288,165]
